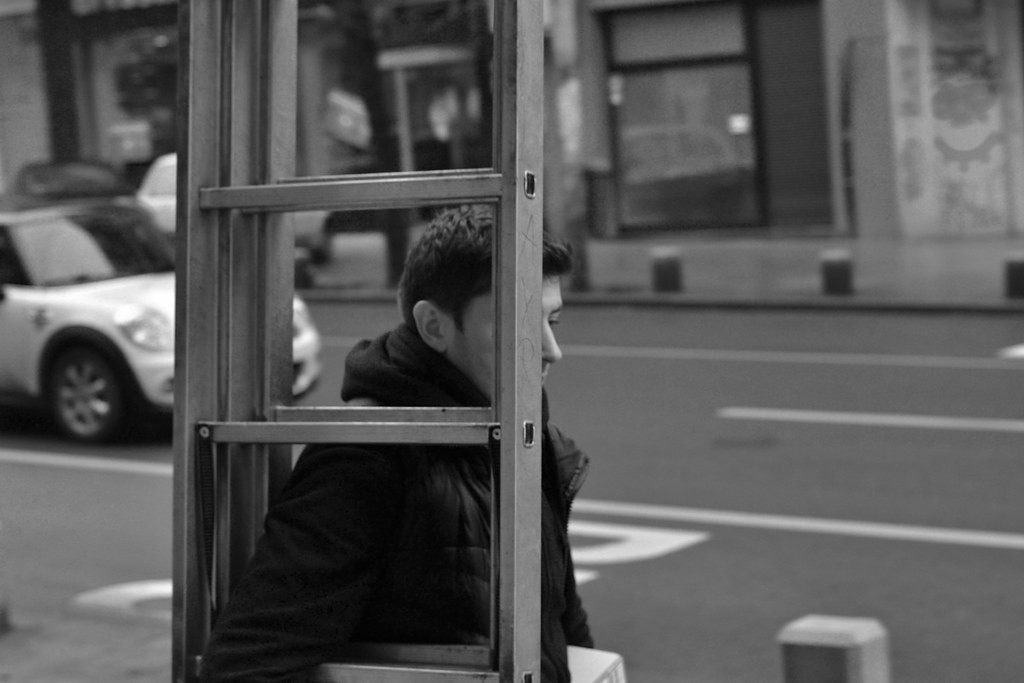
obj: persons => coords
[198,202,595,683]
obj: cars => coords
[0,199,322,443]
[12,151,342,285]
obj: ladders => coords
[170,0,563,683]
